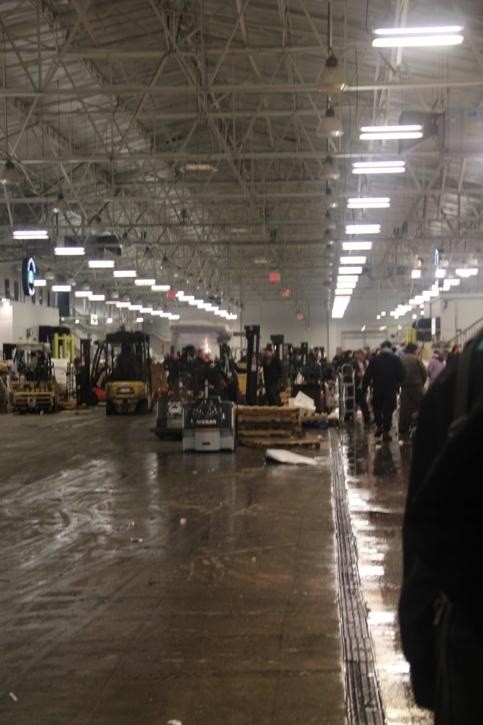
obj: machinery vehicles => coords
[105,330,151,415]
[150,321,238,440]
[7,346,60,412]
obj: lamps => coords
[326,241,372,319]
[345,224,382,235]
[371,25,463,54]
[318,46,342,235]
[357,123,426,142]
[342,197,393,212]
[351,160,410,176]
[10,229,239,323]
[388,257,480,333]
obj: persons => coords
[16,341,461,442]
[399,327,483,725]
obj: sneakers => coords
[374,425,384,436]
[383,432,392,440]
